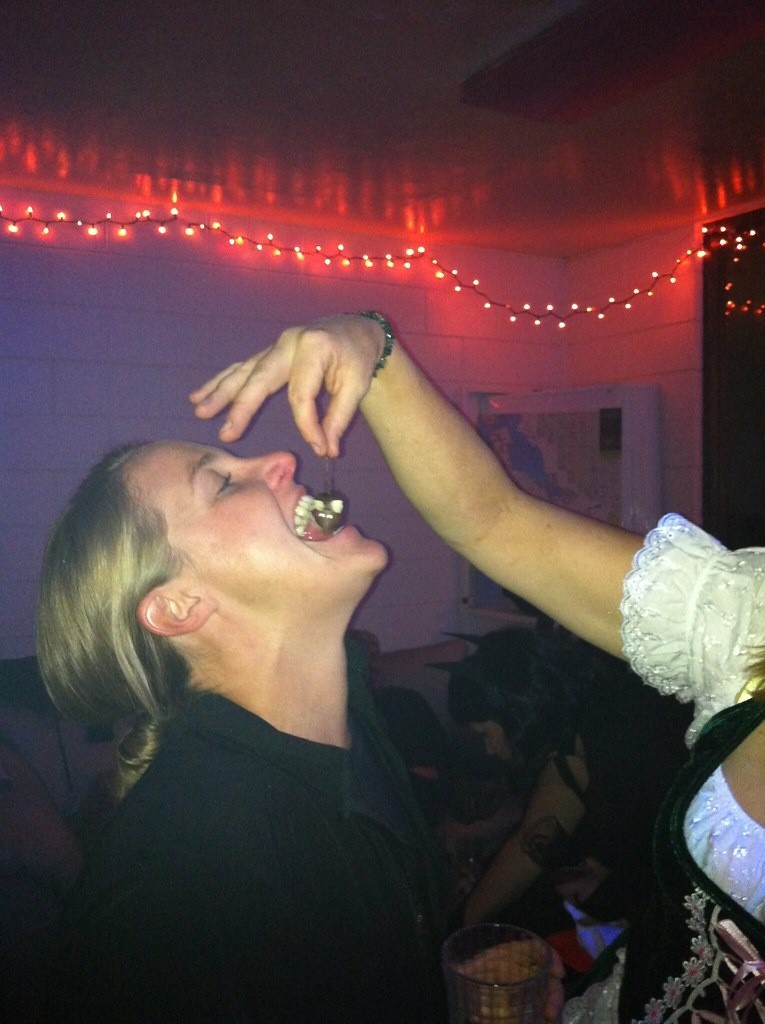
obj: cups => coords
[443,924,557,1024]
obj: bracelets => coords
[360,311,394,379]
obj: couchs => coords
[0,644,495,828]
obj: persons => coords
[342,592,696,935]
[27,438,455,1024]
[189,311,764,1024]
